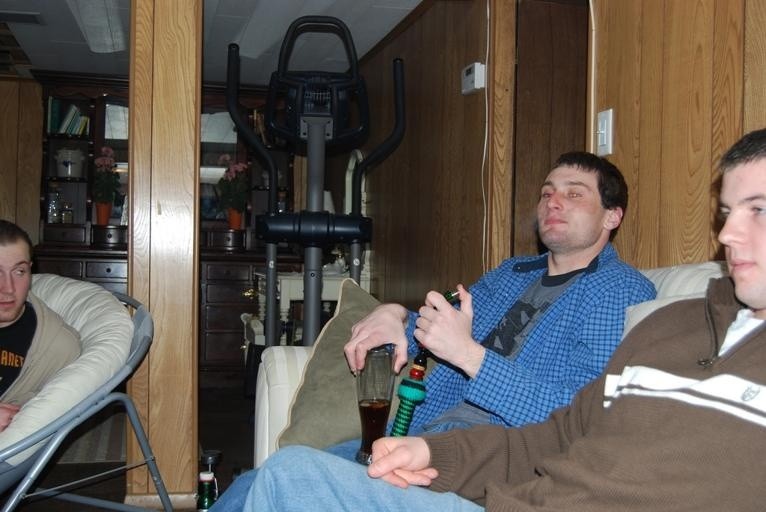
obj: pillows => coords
[276,278,442,451]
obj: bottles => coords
[198,472,215,511]
[47,191,73,225]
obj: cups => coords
[354,349,395,466]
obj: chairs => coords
[0,274,173,512]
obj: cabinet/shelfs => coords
[199,79,299,390]
[30,71,129,307]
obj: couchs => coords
[254,260,729,468]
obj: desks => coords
[253,251,371,347]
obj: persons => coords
[206,148,658,511]
[243,124,765,511]
[0,217,82,433]
